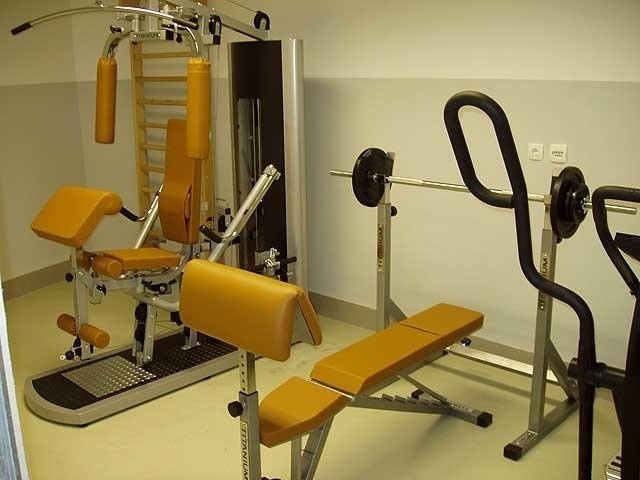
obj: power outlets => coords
[528,144,543,160]
[550,144,567,164]
